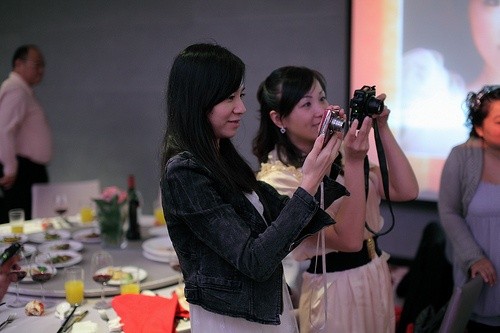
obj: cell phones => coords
[0,242,24,267]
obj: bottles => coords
[127,176,138,201]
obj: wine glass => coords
[91,251,113,309]
[29,249,54,308]
[7,250,29,308]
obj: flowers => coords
[91,188,131,240]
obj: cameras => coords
[317,109,345,146]
[350,88,384,129]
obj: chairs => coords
[32,177,100,226]
[393,220,454,333]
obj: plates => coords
[0,244,36,257]
[72,227,102,242]
[38,239,83,251]
[55,302,74,320]
[0,234,29,244]
[35,250,83,268]
[95,266,147,285]
[17,264,57,281]
[141,225,179,263]
[29,229,72,243]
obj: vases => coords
[97,210,127,248]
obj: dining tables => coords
[1,212,306,333]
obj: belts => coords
[305,234,382,274]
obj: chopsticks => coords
[56,307,88,333]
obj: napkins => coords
[112,289,191,333]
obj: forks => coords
[98,309,110,323]
[0,312,16,331]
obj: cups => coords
[80,199,92,223]
[64,267,84,307]
[9,210,24,233]
[54,192,68,217]
[121,266,140,295]
[153,200,165,224]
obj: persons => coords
[160,44,342,333]
[252,65,416,333]
[0,45,52,225]
[401,0,500,158]
[438,84,500,333]
